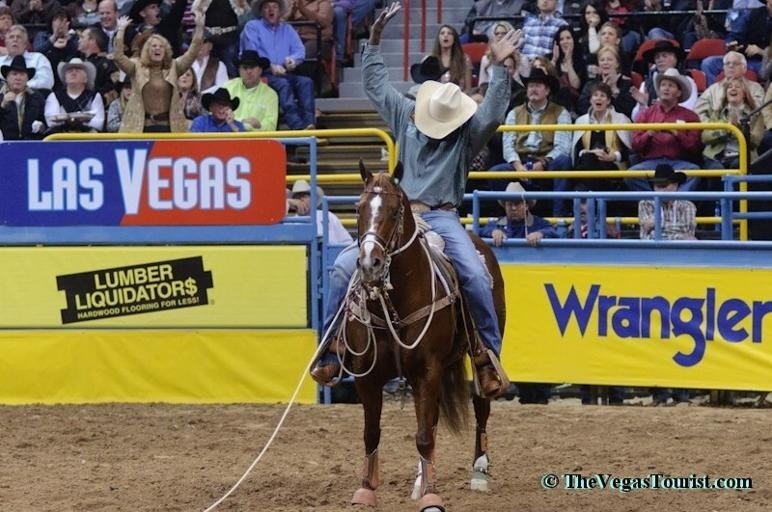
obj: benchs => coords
[460,14,757,95]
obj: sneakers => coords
[304,124,330,147]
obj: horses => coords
[343,158,506,512]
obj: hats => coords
[201,87,240,112]
[413,78,479,140]
[517,67,560,96]
[286,178,327,212]
[231,49,271,71]
[641,39,686,66]
[250,0,290,19]
[128,0,164,24]
[56,57,97,90]
[653,67,693,104]
[647,163,688,191]
[180,28,220,46]
[0,54,36,83]
[409,54,451,84]
[496,181,538,209]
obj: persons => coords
[310,1,526,397]
[286,180,354,246]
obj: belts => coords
[144,111,169,122]
[204,25,241,35]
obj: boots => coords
[472,351,504,396]
[311,353,346,385]
